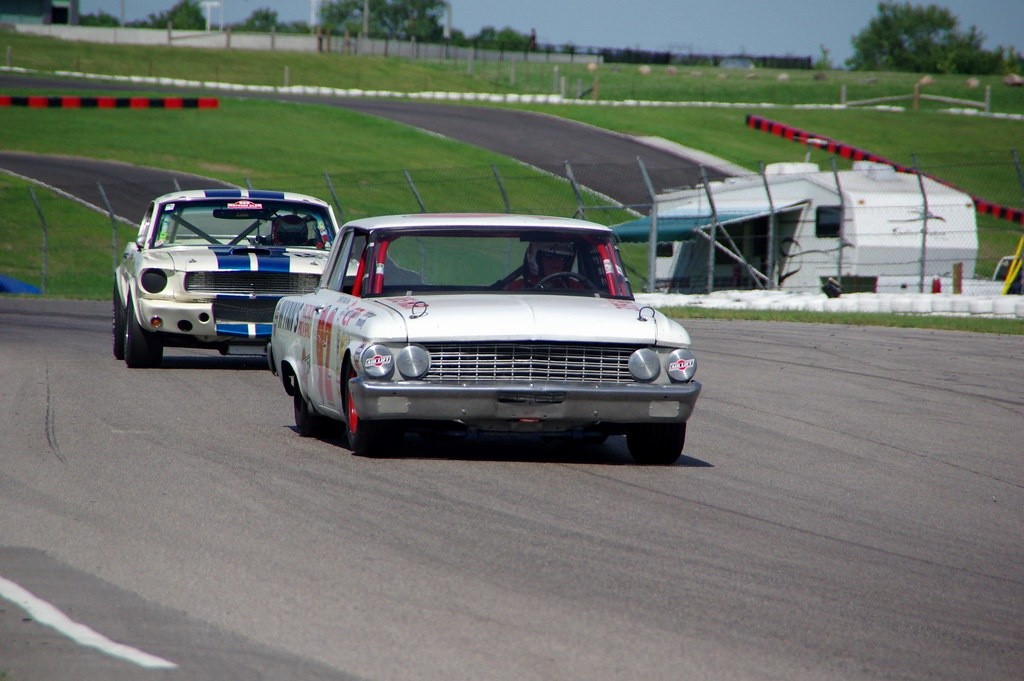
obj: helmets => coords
[271,214,308,248]
[526,240,578,284]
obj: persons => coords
[271,215,309,246]
[504,241,591,296]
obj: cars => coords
[267,210,705,468]
[110,188,372,370]
